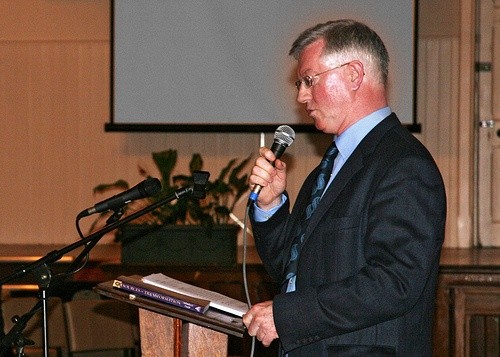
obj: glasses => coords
[295,62,349,90]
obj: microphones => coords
[247,125,296,209]
[77,178,161,218]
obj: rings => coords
[261,330,267,335]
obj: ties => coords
[280,141,340,283]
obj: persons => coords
[243,19,447,357]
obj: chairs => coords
[1,297,141,357]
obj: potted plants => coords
[89,150,253,265]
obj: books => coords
[113,272,250,325]
